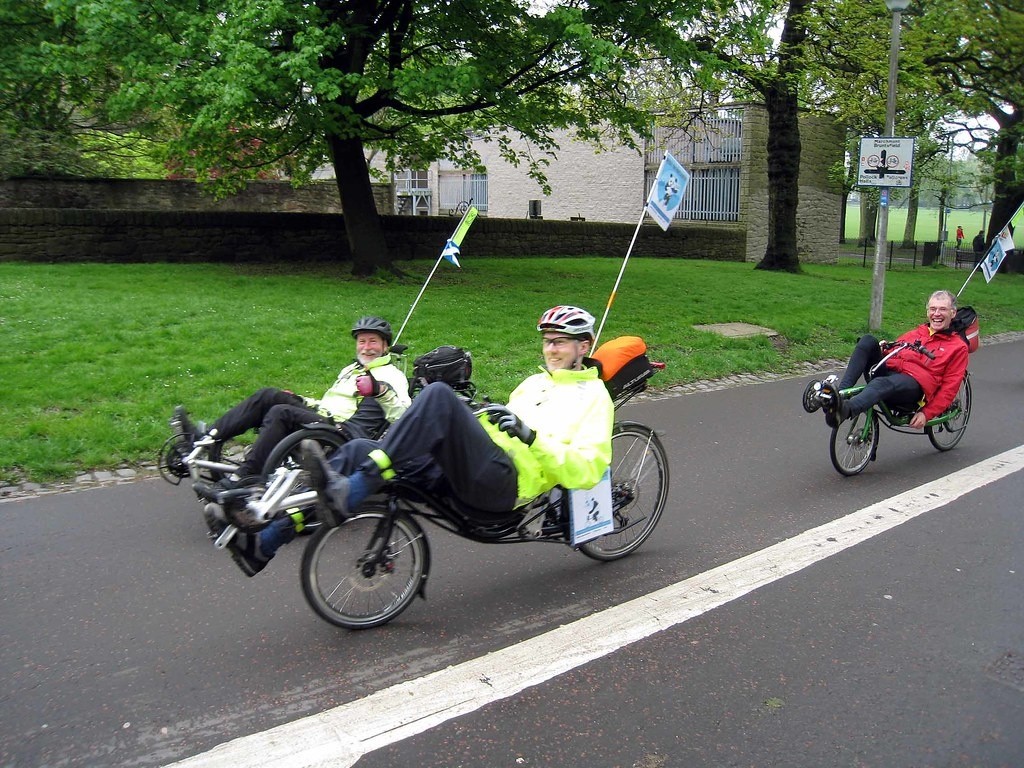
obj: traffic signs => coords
[857,137,915,188]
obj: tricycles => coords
[156,344,672,631]
[803,322,973,477]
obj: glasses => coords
[541,336,585,346]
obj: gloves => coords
[281,389,303,401]
[486,403,536,447]
[356,374,374,396]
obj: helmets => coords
[537,305,595,341]
[350,317,392,337]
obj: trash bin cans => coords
[922,241,939,266]
[1001,249,1024,273]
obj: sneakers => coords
[174,405,207,458]
[300,439,356,527]
[823,380,851,429]
[192,473,243,505]
[202,503,276,578]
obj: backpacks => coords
[412,346,472,382]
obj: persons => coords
[973,230,986,272]
[822,291,969,429]
[205,306,614,578]
[954,226,965,253]
[173,317,412,502]
